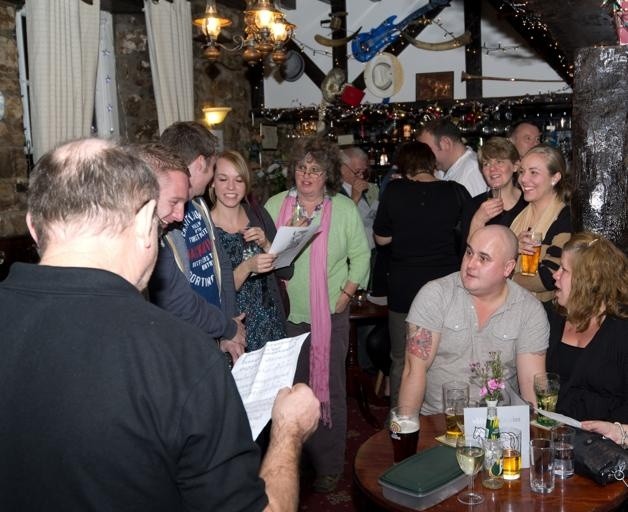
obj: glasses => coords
[294,167,325,180]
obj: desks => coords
[344,288,388,406]
[352,405,628,512]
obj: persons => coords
[374,143,477,425]
[414,118,491,197]
[144,121,247,369]
[209,150,294,351]
[329,147,376,283]
[509,146,581,317]
[265,135,371,494]
[508,124,539,158]
[0,136,320,512]
[398,225,551,414]
[553,232,628,448]
[378,149,405,199]
[115,142,190,301]
[467,135,529,239]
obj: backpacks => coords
[244,192,290,321]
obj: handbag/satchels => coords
[564,423,628,488]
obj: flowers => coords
[470,350,506,400]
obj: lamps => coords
[198,1,297,68]
[195,106,235,131]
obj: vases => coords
[482,401,505,489]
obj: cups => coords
[290,203,305,228]
[500,427,523,481]
[529,437,556,496]
[486,185,501,199]
[551,425,576,483]
[238,225,253,257]
[387,404,420,466]
[480,435,504,491]
[533,374,561,427]
[441,379,470,442]
[518,231,542,278]
[455,431,485,507]
